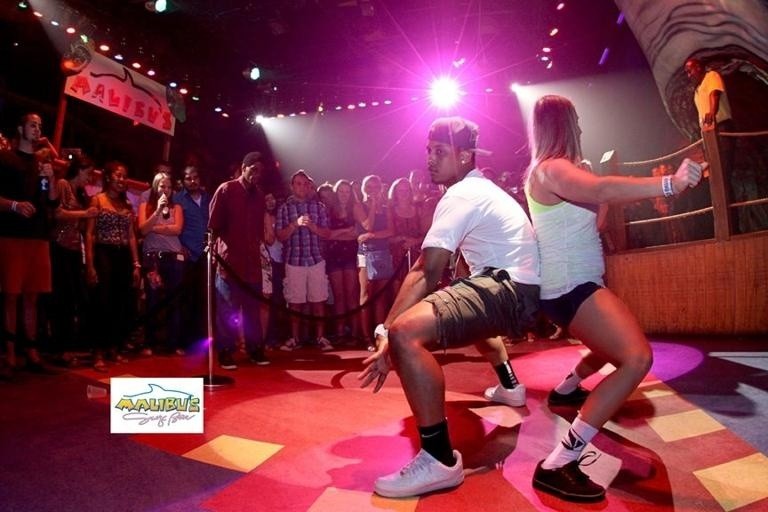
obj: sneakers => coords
[280,335,303,351]
[374,448,464,498]
[485,384,525,406]
[250,349,271,365]
[315,336,333,350]
[548,386,591,406]
[532,451,604,501]
[218,349,237,370]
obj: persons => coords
[1,112,457,383]
[482,164,562,342]
[684,55,742,236]
[523,94,709,501]
[356,115,543,500]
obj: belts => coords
[145,251,184,261]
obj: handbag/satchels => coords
[367,250,392,281]
[271,262,285,297]
[46,204,76,242]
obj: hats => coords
[429,116,479,150]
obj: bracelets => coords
[372,325,388,341]
[661,174,674,199]
